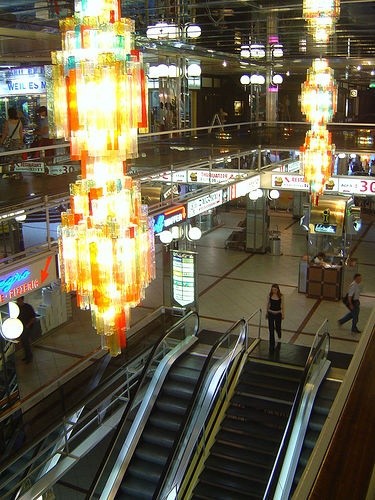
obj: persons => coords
[0,105,57,183]
[265,284,285,349]
[337,274,362,333]
[311,252,325,263]
[153,102,174,139]
[16,296,38,365]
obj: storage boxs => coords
[36,282,67,335]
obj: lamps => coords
[157,223,202,246]
[297,56,337,206]
[147,56,202,81]
[302,0,340,43]
[240,74,283,93]
[0,301,24,344]
[46,0,156,357]
[247,188,280,202]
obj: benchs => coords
[225,218,247,252]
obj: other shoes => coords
[26,358,32,364]
[338,320,341,327]
[352,328,361,333]
[22,357,28,361]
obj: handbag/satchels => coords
[342,293,356,312]
